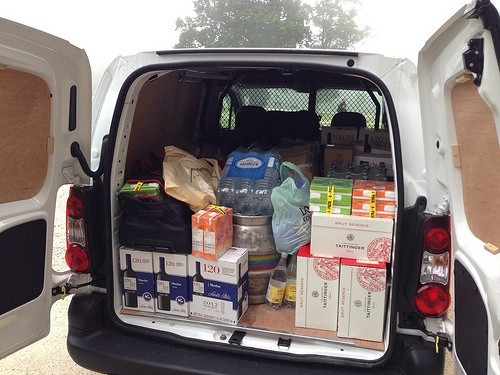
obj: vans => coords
[1,0,500,375]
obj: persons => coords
[337,98,347,112]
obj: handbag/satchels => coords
[270,161,312,256]
[164,146,223,211]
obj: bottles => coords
[217,144,282,215]
[283,255,297,308]
[327,160,388,182]
[192,262,204,296]
[266,252,288,308]
[123,254,137,307]
[156,257,170,310]
[238,263,243,301]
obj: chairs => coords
[220,106,366,155]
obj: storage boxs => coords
[120,181,249,326]
[295,177,395,343]
[278,126,394,182]
